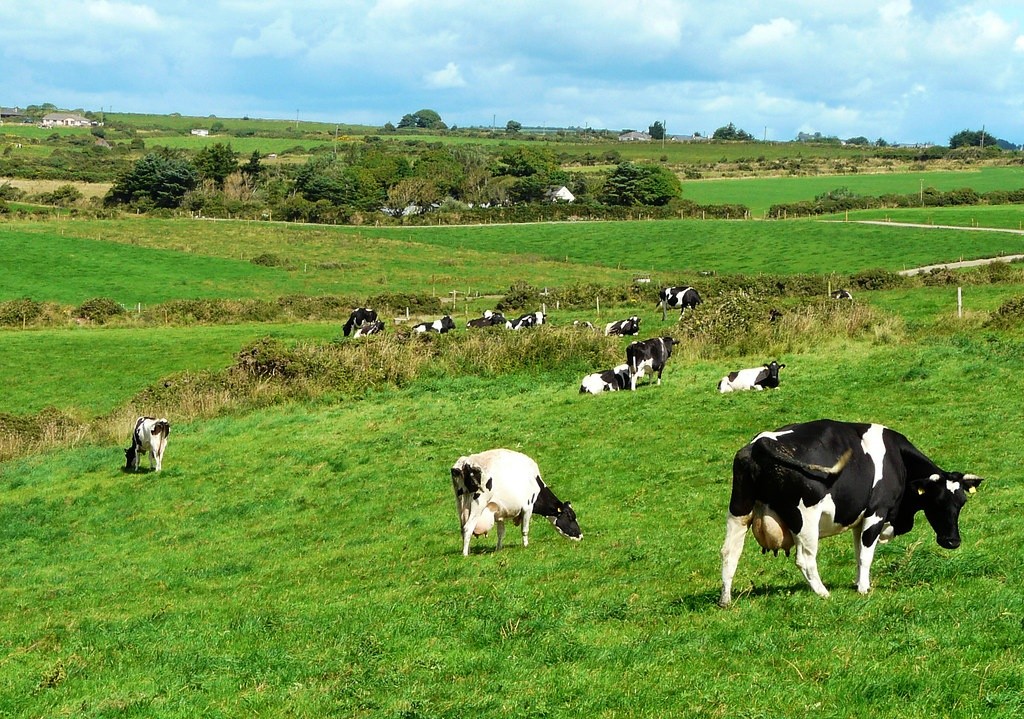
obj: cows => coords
[124,416,170,472]
[451,448,583,556]
[579,337,679,395]
[719,419,987,606]
[718,361,786,394]
[342,306,548,338]
[701,271,724,296]
[768,281,852,325]
[573,287,703,337]
